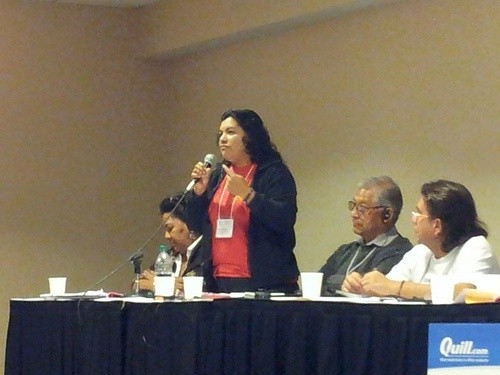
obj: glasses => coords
[410,212,443,223]
[348,201,391,215]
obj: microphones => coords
[186,154,217,193]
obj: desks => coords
[4,294,500,375]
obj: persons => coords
[183,108,300,295]
[318,175,414,297]
[342,180,500,304]
[131,191,208,295]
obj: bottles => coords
[154,244,174,299]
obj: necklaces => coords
[345,246,377,276]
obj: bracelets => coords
[243,187,253,201]
[398,280,405,297]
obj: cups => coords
[183,276,203,300]
[299,272,323,300]
[49,276,66,296]
[430,273,456,304]
